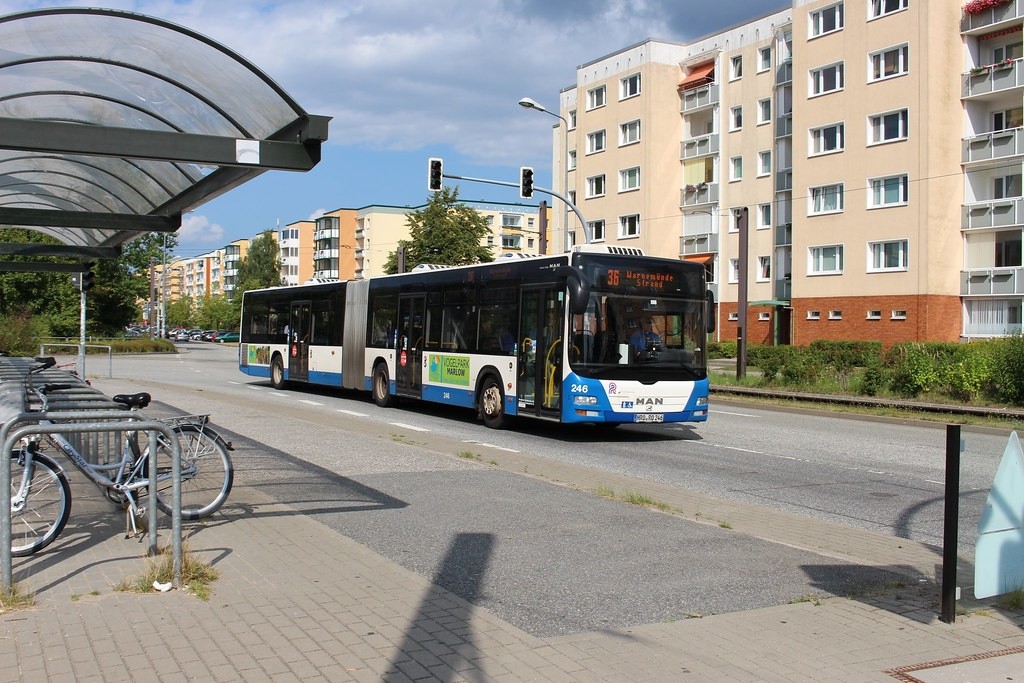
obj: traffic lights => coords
[79,260,96,292]
[520,167,534,199]
[427,157,443,191]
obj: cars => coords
[124,323,233,342]
[214,331,240,343]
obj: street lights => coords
[518,97,568,254]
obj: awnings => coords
[677,63,714,87]
[683,254,713,275]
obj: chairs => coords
[498,326,606,364]
[253,321,278,335]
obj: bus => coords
[237,243,714,431]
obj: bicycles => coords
[8,358,236,559]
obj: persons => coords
[284,315,296,334]
[629,318,662,357]
[256,346,267,364]
